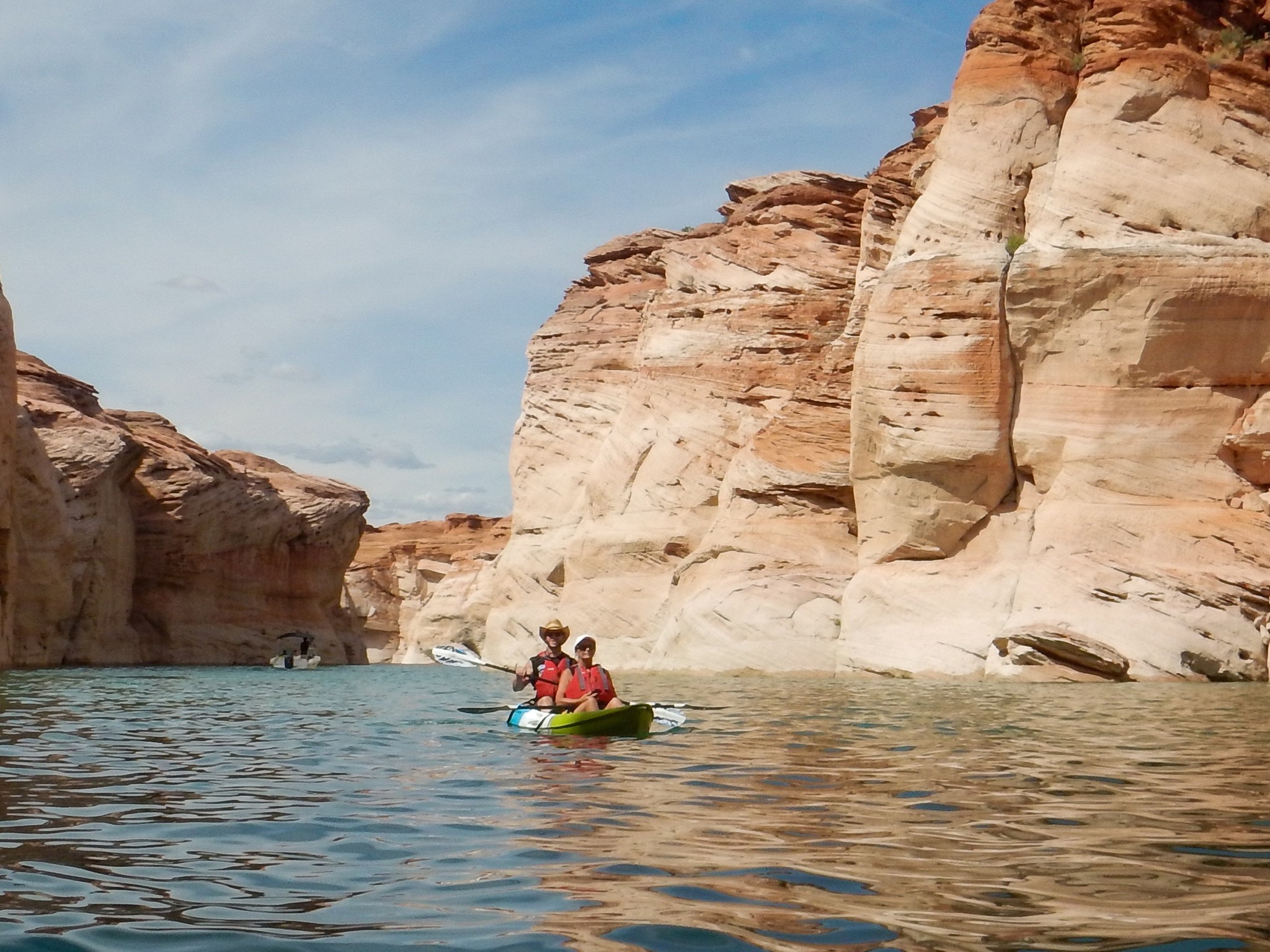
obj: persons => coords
[513,619,578,713]
[279,650,288,656]
[300,637,315,659]
[555,634,626,714]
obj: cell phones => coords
[588,688,602,699]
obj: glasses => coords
[576,644,595,651]
[544,631,565,637]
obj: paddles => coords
[430,643,559,686]
[456,702,574,714]
[617,699,730,711]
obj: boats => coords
[505,702,654,740]
[269,655,321,669]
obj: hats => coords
[540,619,570,644]
[575,635,596,653]
